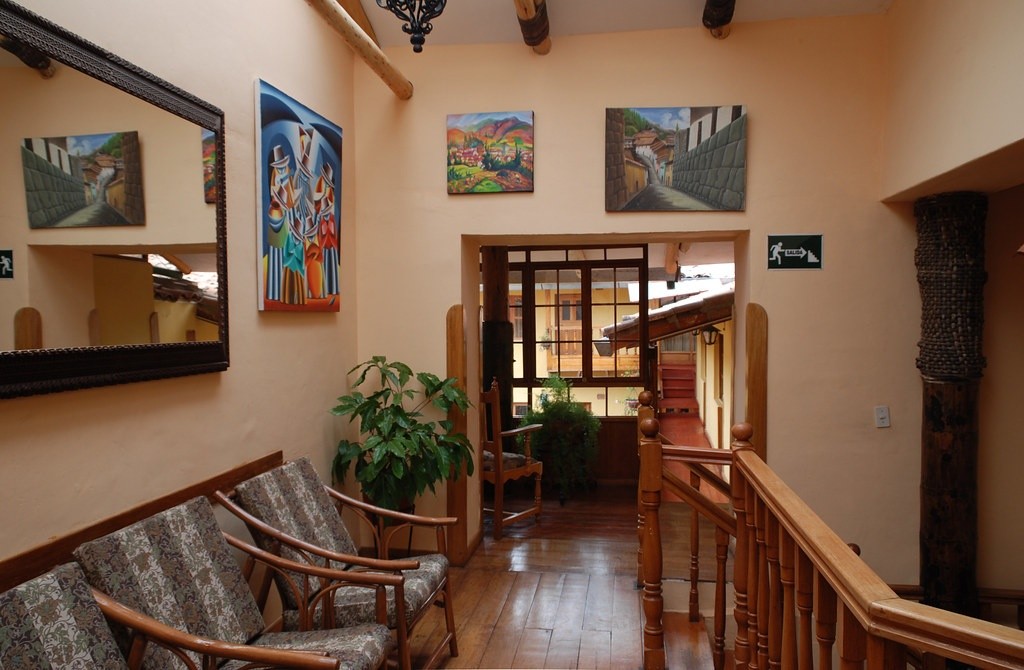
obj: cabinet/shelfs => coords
[482,319,513,498]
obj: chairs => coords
[478,376,542,539]
[0,450,461,670]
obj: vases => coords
[626,398,641,409]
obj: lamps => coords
[691,325,719,345]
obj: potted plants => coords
[510,376,603,502]
[330,353,474,527]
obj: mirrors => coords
[0,0,231,401]
